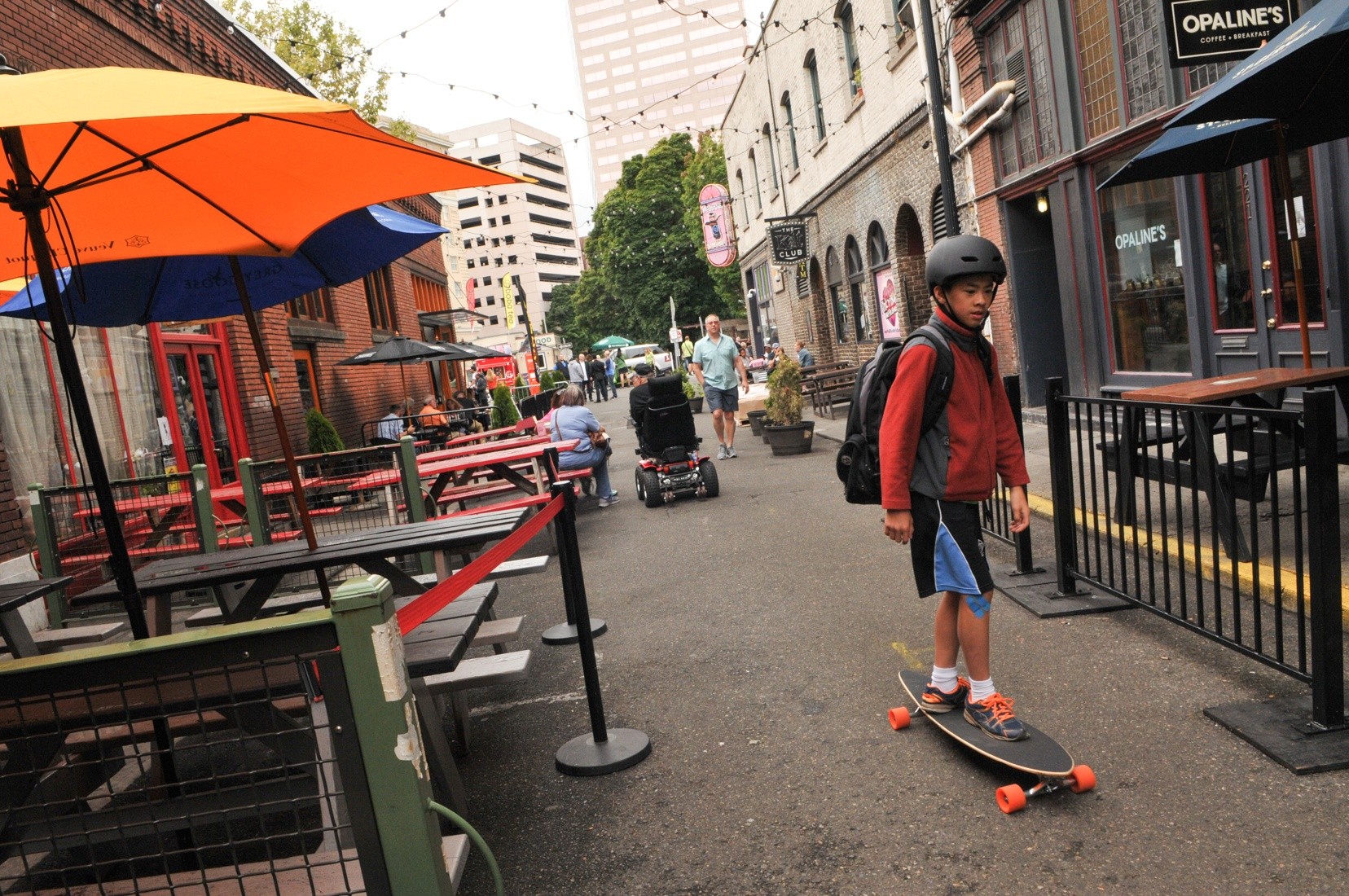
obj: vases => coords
[747,409,771,445]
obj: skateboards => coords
[885,662,1096,818]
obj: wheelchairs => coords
[635,373,719,508]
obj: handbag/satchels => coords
[588,431,611,449]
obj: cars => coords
[608,344,672,376]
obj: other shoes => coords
[688,373,693,376]
[611,490,618,496]
[594,401,601,403]
[727,446,738,458]
[626,385,631,388]
[611,396,617,399]
[590,398,593,401]
[620,386,624,389]
[717,442,727,459]
[598,496,621,507]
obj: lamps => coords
[1035,191,1048,213]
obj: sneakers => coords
[921,677,971,712]
[963,692,1031,742]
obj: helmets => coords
[924,234,1007,297]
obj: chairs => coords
[641,374,699,456]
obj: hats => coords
[772,343,779,348]
[764,344,771,348]
[627,362,655,379]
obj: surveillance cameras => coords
[748,294,753,300]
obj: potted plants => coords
[853,70,863,96]
[763,347,814,456]
[682,381,704,414]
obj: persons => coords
[692,313,750,460]
[680,335,695,375]
[732,336,815,379]
[536,384,619,507]
[377,366,498,448]
[627,363,656,422]
[644,349,656,371]
[615,348,630,388]
[554,349,618,403]
[879,234,1032,742]
[1213,242,1236,328]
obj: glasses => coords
[707,321,720,325]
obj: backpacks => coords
[836,325,994,505]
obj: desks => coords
[414,435,550,512]
[1113,367,1349,563]
[445,426,516,446]
[66,507,528,619]
[0,576,74,659]
[347,439,580,518]
[72,475,322,540]
[0,580,499,742]
[801,360,860,418]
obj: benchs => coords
[185,555,550,627]
[132,506,342,540]
[0,835,470,896]
[801,381,855,395]
[1097,418,1253,453]
[0,622,125,654]
[65,649,531,746]
[1217,440,1349,476]
[469,615,524,646]
[314,478,361,486]
[397,462,592,522]
[60,529,302,566]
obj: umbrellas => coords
[0,205,447,613]
[0,67,538,788]
[332,330,460,428]
[446,338,512,399]
[385,333,494,412]
[591,336,635,349]
[1093,0,1349,367]
